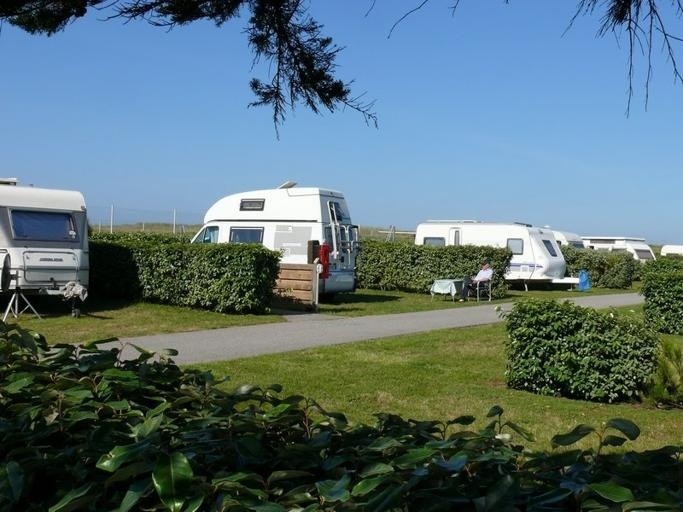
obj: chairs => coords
[467,268,492,302]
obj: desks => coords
[430,278,464,302]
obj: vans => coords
[189,182,359,292]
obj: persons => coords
[454,261,493,304]
